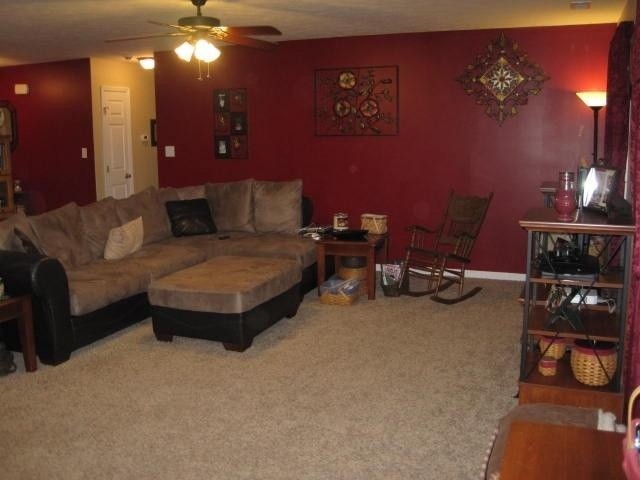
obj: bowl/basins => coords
[381,281,400,297]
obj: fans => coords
[104,1,283,51]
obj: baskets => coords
[319,290,359,305]
[570,339,618,386]
[361,214,387,234]
[539,336,566,360]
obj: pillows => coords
[26,200,92,271]
[116,184,169,245]
[254,178,304,234]
[158,185,206,235]
[205,179,254,232]
[78,196,120,261]
[104,215,144,259]
[0,209,35,255]
[164,198,218,238]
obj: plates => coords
[331,229,369,239]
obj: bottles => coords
[554,172,577,221]
[13,178,24,193]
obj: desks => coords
[486,417,627,480]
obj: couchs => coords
[0,193,334,366]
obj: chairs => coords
[397,188,493,304]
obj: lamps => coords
[175,34,221,64]
[576,91,607,163]
[136,57,155,69]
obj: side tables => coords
[315,229,391,300]
[0,286,37,372]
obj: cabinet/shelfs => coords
[519,205,637,425]
[536,180,584,258]
[212,87,249,160]
[0,99,18,223]
